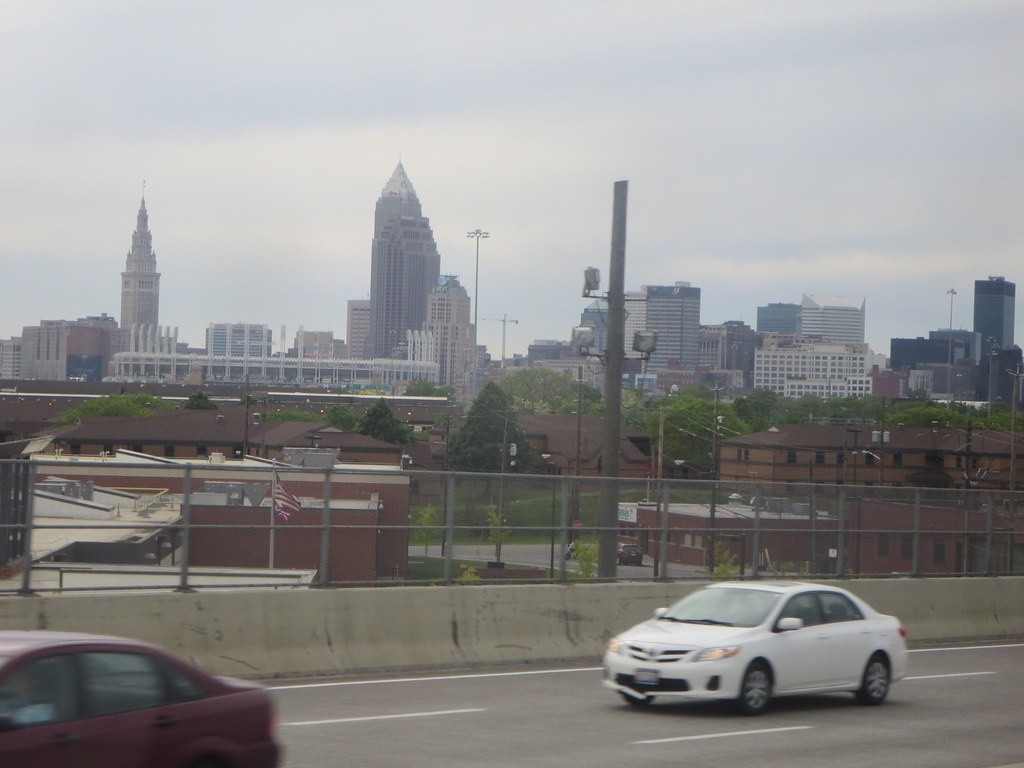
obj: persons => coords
[569,541,576,552]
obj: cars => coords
[599,577,907,715]
[1,630,282,768]
[616,542,643,565]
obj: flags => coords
[274,500,289,521]
[274,473,300,511]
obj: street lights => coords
[730,493,760,579]
[542,454,569,581]
[571,266,660,583]
[838,451,858,579]
[946,288,957,412]
[862,450,884,501]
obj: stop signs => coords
[573,520,582,527]
